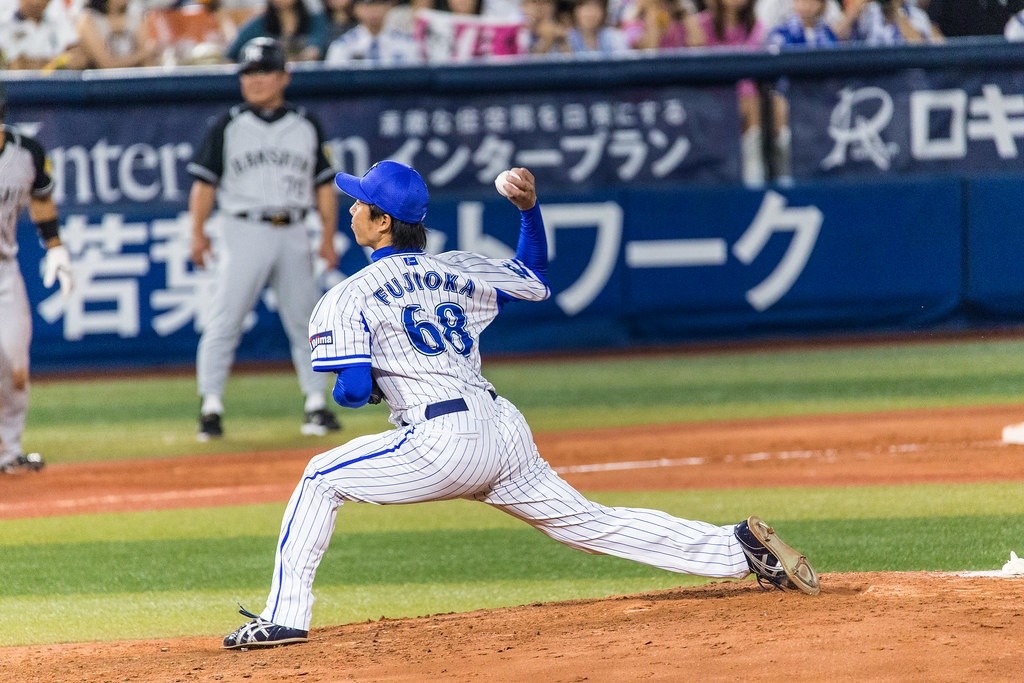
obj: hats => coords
[335,158,429,223]
[234,35,286,76]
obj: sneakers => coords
[222,602,308,648]
[0,454,44,471]
[735,516,821,597]
[193,395,223,439]
[301,409,341,436]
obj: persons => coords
[185,38,340,441]
[0,130,71,478]
[222,159,820,649]
[0,0,945,191]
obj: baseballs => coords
[495,169,522,197]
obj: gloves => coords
[42,244,74,300]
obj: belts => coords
[400,389,496,427]
[237,211,308,225]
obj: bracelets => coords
[32,217,59,244]
